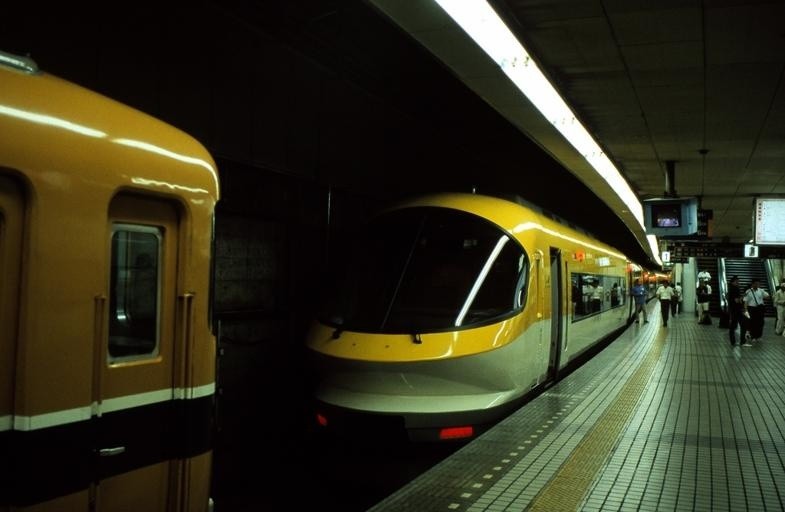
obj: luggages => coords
[702,311,712,324]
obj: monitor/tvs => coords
[645,199,697,235]
[752,197,785,248]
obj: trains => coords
[297,185,672,453]
[0,46,228,511]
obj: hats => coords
[730,275,740,282]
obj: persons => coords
[728,275,769,347]
[656,280,683,327]
[611,282,623,307]
[773,278,785,336]
[572,278,604,318]
[631,278,650,323]
[696,267,712,324]
